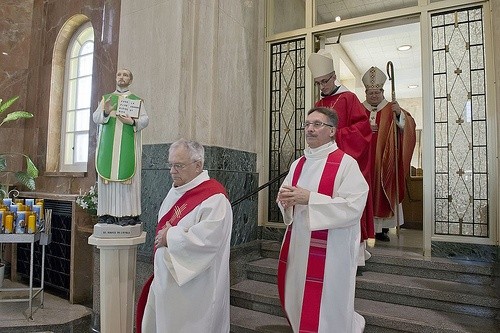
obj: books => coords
[116,97,141,119]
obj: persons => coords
[307,49,375,277]
[93,69,149,225]
[276,106,369,333]
[362,66,416,242]
[136,136,233,333]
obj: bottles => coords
[16,202,23,211]
[4,214,13,233]
[36,200,44,220]
[28,214,36,234]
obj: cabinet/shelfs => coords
[0,222,45,321]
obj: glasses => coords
[168,161,197,170]
[301,121,332,128]
[366,91,382,97]
[313,75,332,87]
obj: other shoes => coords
[375,232,390,242]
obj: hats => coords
[306,54,334,78]
[362,67,387,90]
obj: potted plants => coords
[0,95,38,288]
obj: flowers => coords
[75,185,98,215]
